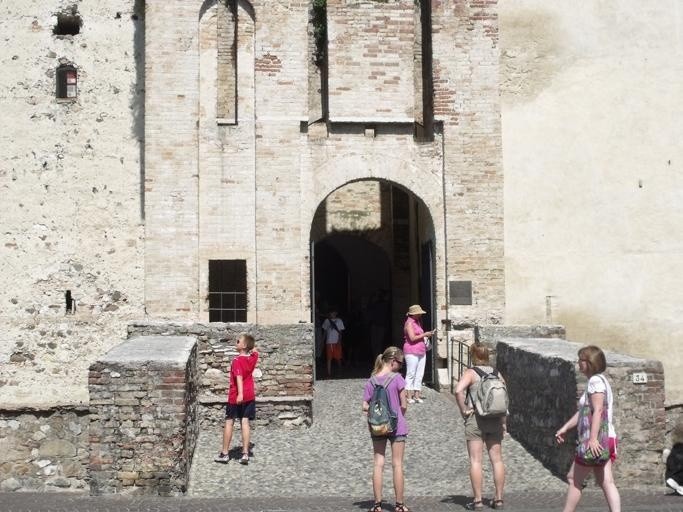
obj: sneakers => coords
[239,453,249,465]
[215,453,230,462]
[406,397,424,405]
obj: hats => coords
[408,305,427,315]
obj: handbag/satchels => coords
[424,337,432,351]
[578,403,610,466]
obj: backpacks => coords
[470,366,509,417]
[367,374,398,436]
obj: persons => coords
[665,467,683,496]
[315,289,328,381]
[554,344,622,512]
[454,342,510,512]
[362,345,411,512]
[402,304,438,405]
[321,307,346,376]
[213,332,259,462]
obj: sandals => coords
[368,502,383,512]
[394,502,412,512]
[466,500,484,511]
[487,498,503,510]
